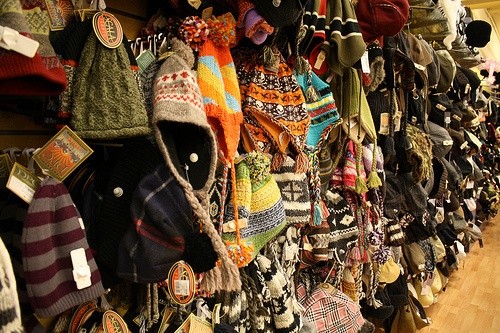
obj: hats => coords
[209,164,233,231]
[236,43,310,154]
[140,42,176,128]
[106,265,301,333]
[0,237,25,333]
[245,0,500,333]
[26,319,105,333]
[56,16,147,134]
[151,38,212,130]
[22,175,106,318]
[0,0,67,95]
[91,137,216,285]
[71,28,152,138]
[36,28,72,146]
[197,12,244,163]
[223,154,286,267]
